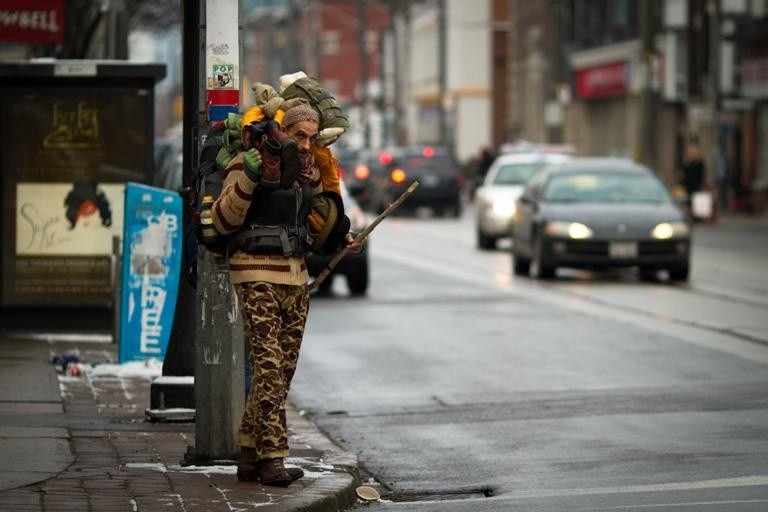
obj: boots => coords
[237,447,304,487]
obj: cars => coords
[333,144,461,217]
[304,179,368,298]
[475,142,689,281]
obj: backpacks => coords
[196,71,352,254]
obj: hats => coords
[305,191,350,255]
[282,105,320,127]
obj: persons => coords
[476,145,494,173]
[211,102,367,487]
[683,147,706,225]
